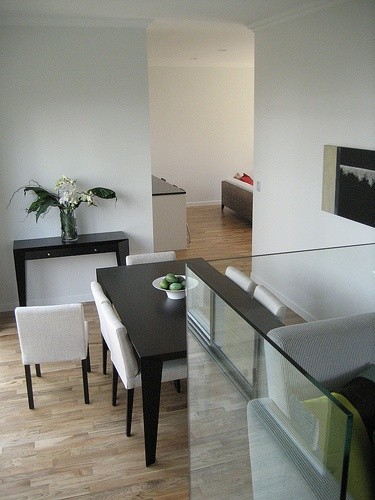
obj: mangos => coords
[161,273,185,290]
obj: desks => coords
[96,257,286,467]
[152,175,188,252]
[13,231,129,307]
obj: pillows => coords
[234,173,253,185]
[299,363,375,500]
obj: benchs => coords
[220,177,253,225]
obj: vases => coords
[59,209,79,242]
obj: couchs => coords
[246,313,375,500]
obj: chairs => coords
[15,303,91,410]
[90,252,286,437]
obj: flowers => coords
[3,175,117,240]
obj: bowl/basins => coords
[151,275,199,299]
